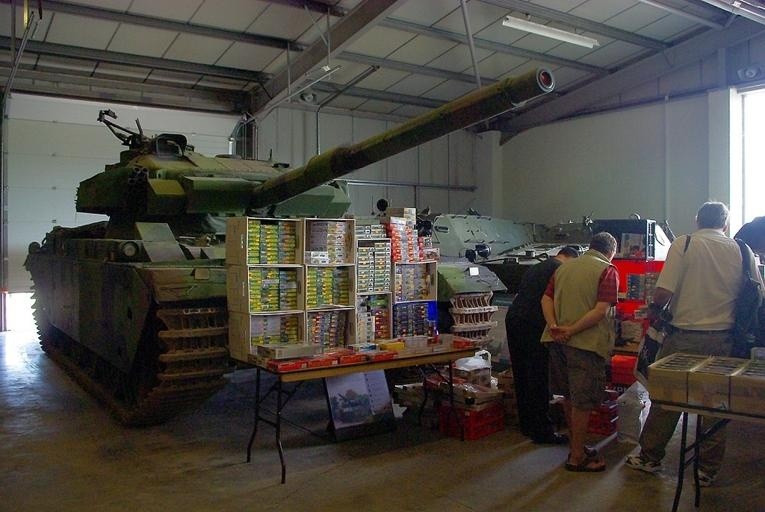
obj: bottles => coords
[427,319,439,343]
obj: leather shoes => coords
[538,433,568,444]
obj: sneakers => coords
[694,468,720,487]
[625,455,662,472]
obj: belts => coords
[671,326,731,334]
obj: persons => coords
[541,232,618,472]
[503,247,579,447]
[625,201,764,487]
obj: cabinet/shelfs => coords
[225,217,438,353]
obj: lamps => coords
[501,8,600,49]
[701,0,765,25]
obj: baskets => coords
[440,405,504,441]
[564,396,617,436]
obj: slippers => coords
[567,447,605,472]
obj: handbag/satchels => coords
[734,237,765,359]
[633,326,666,386]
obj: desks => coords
[230,347,482,484]
[652,402,765,512]
